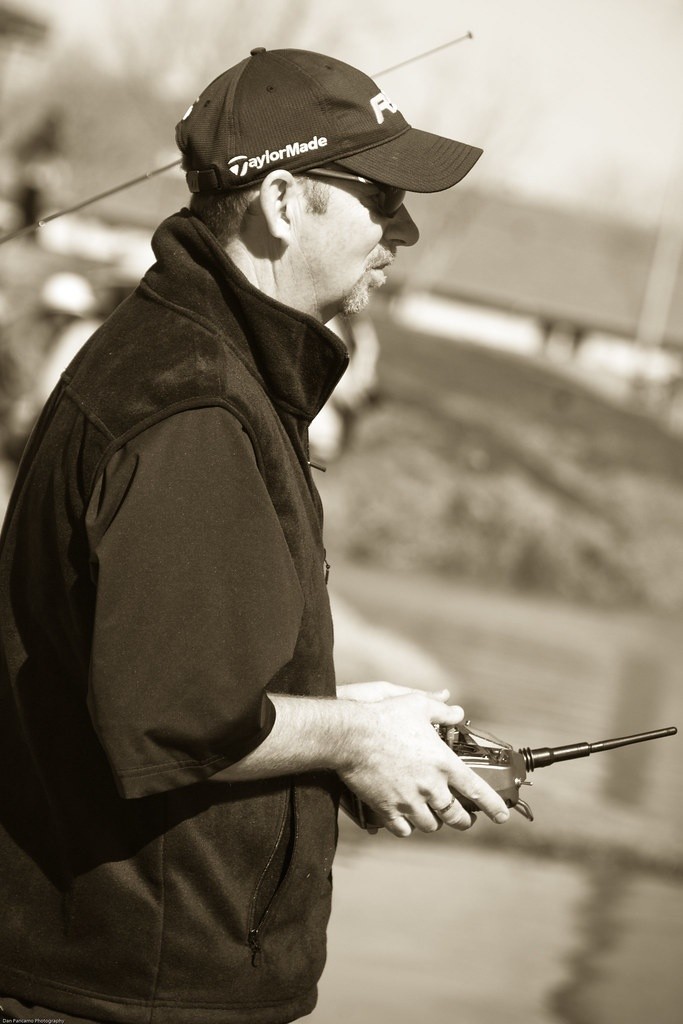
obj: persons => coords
[299,309,380,464]
[0,256,165,471]
[0,47,517,1023]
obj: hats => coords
[175,46,483,193]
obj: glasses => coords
[302,166,407,218]
